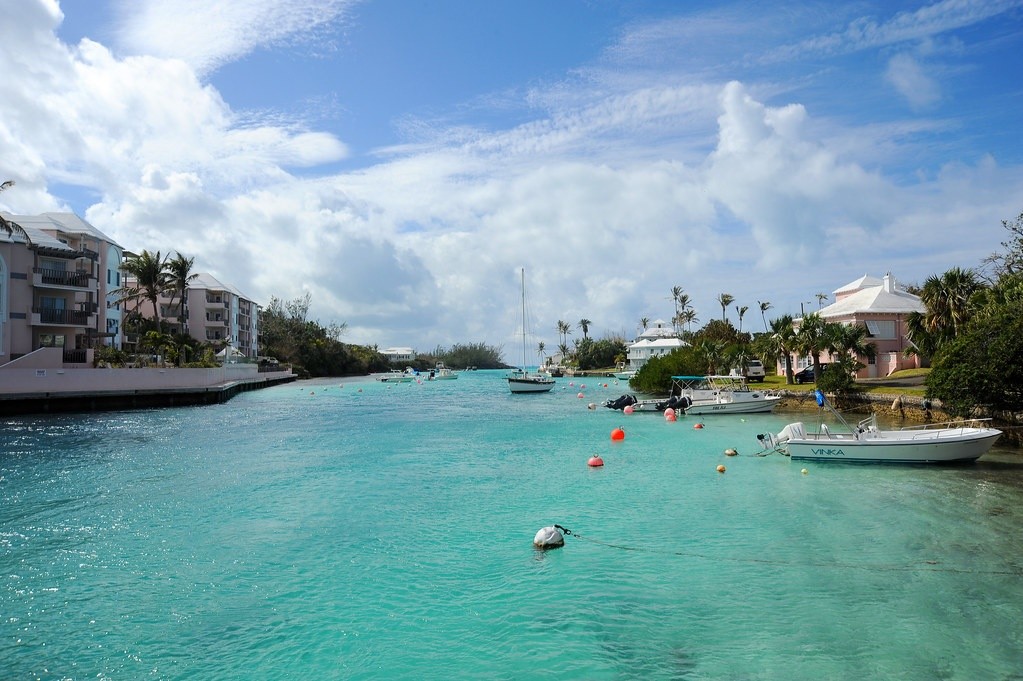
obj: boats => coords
[552,368,564,377]
[376,374,413,382]
[612,372,636,380]
[605,376,782,415]
[757,390,1003,463]
[426,369,459,382]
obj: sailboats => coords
[507,268,557,393]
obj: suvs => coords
[729,360,765,383]
[795,363,826,384]
